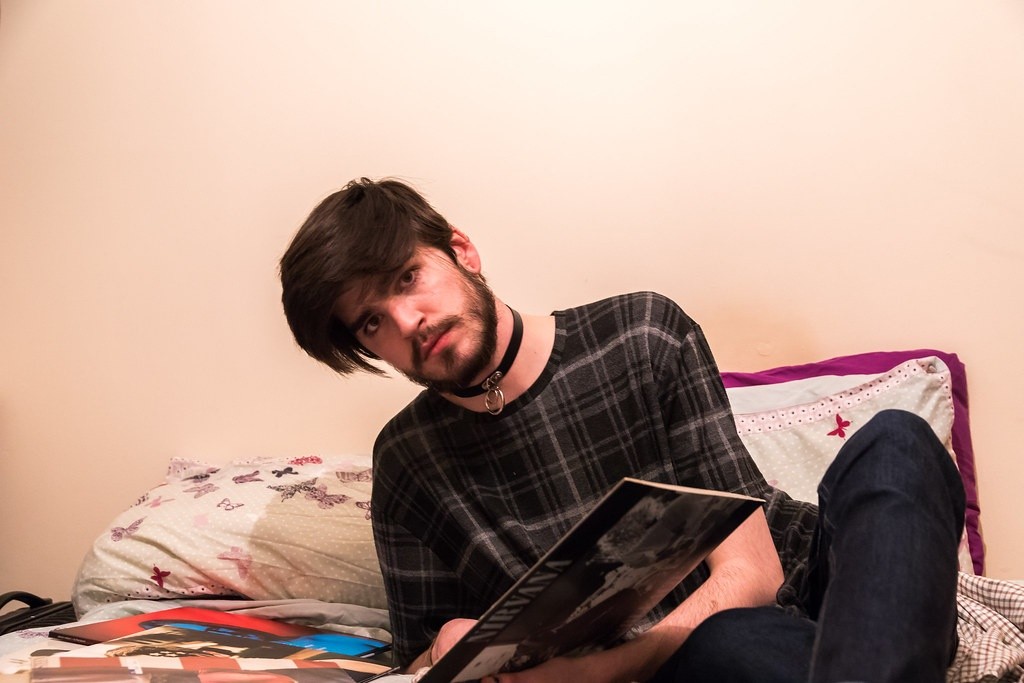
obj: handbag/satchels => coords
[0,591,77,636]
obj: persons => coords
[280,180,966,683]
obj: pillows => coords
[73,348,987,599]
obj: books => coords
[0,606,399,683]
[368,477,767,683]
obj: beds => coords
[0,598,1024,683]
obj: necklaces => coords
[450,303,523,415]
